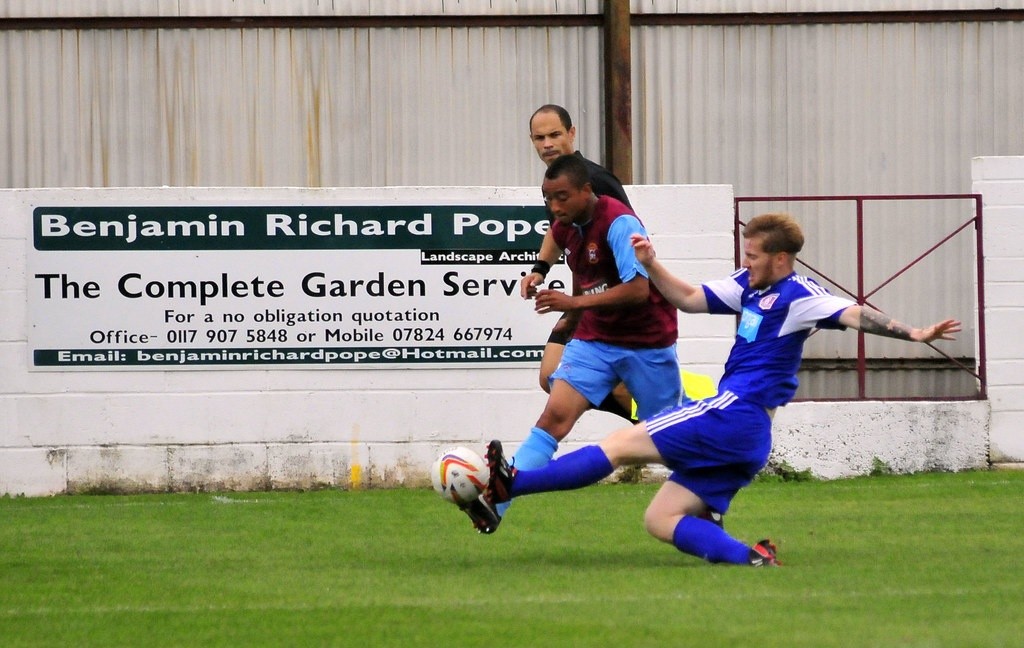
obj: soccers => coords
[429,446,490,505]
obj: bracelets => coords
[531,260,551,279]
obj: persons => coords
[484,210,963,565]
[457,154,696,535]
[529,105,645,485]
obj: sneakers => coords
[751,541,781,566]
[459,491,501,534]
[696,504,724,530]
[483,440,519,506]
